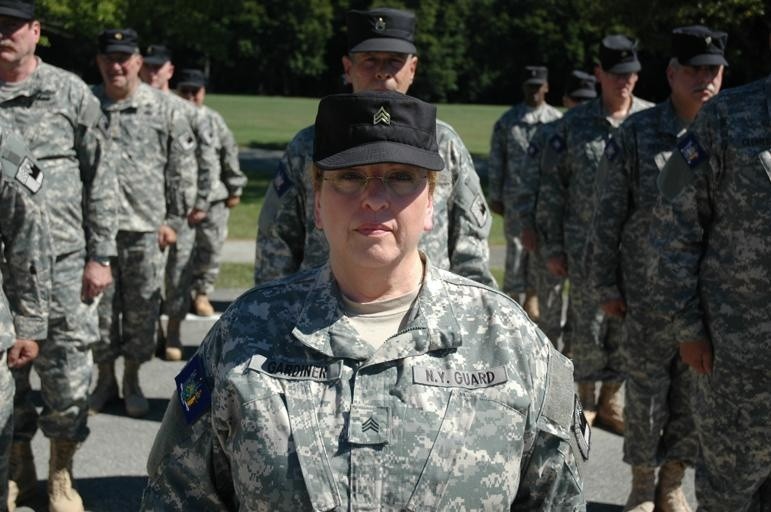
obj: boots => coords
[576,380,625,435]
[524,292,541,322]
[6,438,84,512]
[164,318,182,361]
[194,289,214,315]
[623,460,691,512]
[89,362,148,415]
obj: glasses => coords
[320,171,427,197]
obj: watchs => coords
[94,255,113,267]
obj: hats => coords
[97,28,206,88]
[312,90,444,170]
[522,66,548,84]
[567,34,642,99]
[347,9,417,54]
[0,0,35,20]
[672,26,728,66]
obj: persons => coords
[0,120,53,512]
[588,25,729,512]
[532,34,659,435]
[173,69,248,318]
[657,79,771,511]
[485,65,600,360]
[253,8,499,295]
[136,43,215,362]
[89,27,199,424]
[138,90,593,512]
[0,0,121,512]
